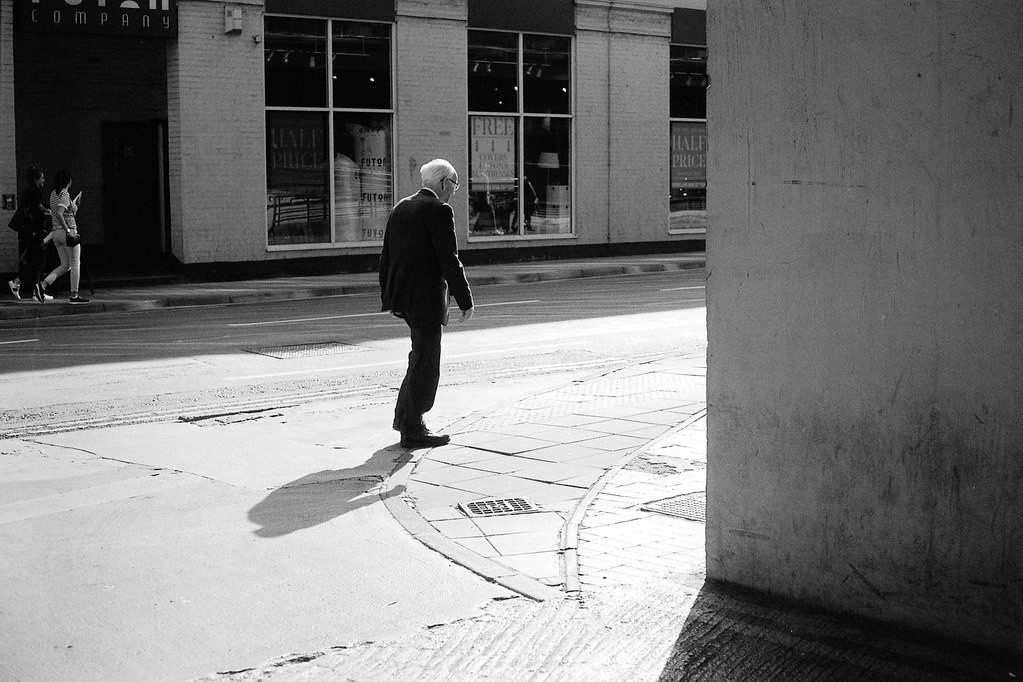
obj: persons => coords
[8,167,90,303]
[469,162,538,235]
[378,158,474,447]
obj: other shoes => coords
[400,433,449,448]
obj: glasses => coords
[441,177,461,191]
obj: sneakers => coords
[8,281,21,301]
[35,282,45,303]
[69,295,90,305]
[32,292,53,302]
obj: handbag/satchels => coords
[66,234,81,247]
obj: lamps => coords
[265,51,316,67]
[537,67,544,77]
[487,63,493,72]
[525,66,532,75]
[473,63,479,72]
